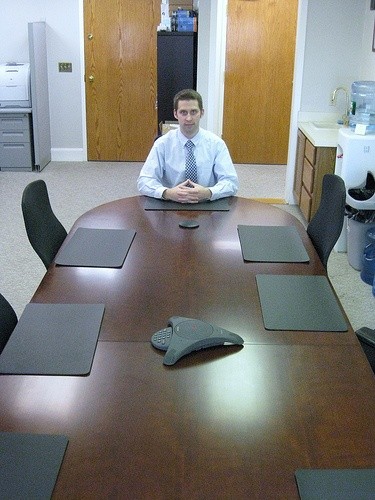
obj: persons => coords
[137,89,239,203]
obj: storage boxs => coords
[175,9,198,31]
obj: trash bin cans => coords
[346,168,375,271]
[358,227,375,286]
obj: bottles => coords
[348,80,375,132]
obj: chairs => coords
[21,182,67,270]
[0,294,18,355]
[306,175,346,270]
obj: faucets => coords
[331,86,350,126]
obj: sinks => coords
[308,120,344,131]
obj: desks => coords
[1,197,374,500]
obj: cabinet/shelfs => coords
[0,114,33,172]
[294,130,316,222]
[156,31,197,138]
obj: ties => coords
[184,140,197,189]
